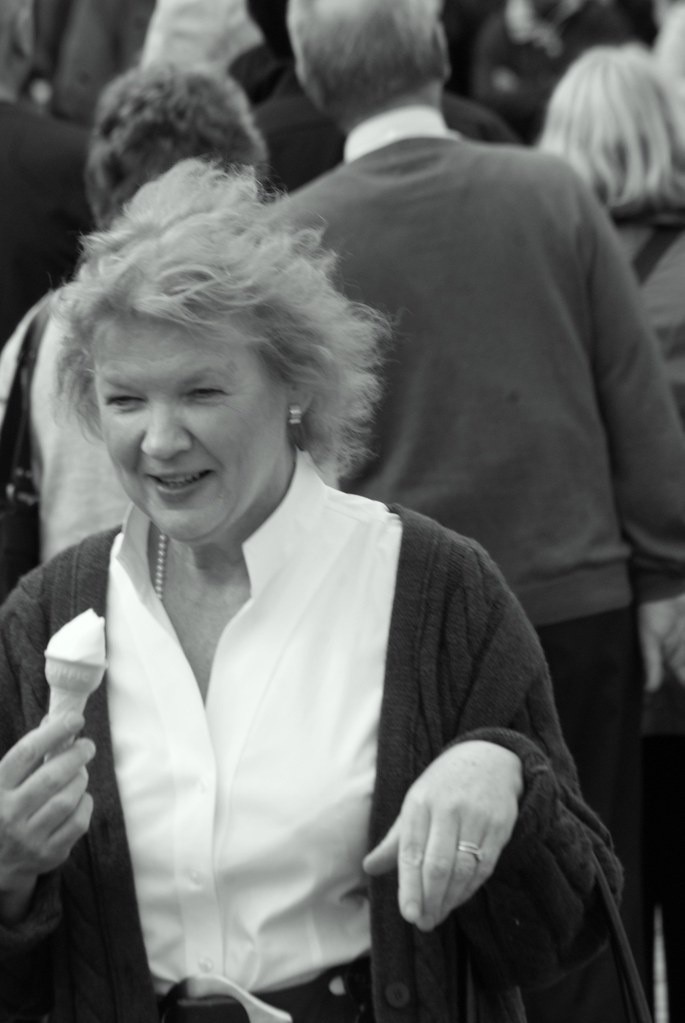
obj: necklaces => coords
[155,535,166,598]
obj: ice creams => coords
[43,608,108,757]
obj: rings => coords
[458,841,482,860]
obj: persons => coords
[277,0,685,986]
[439,0,684,431]
[0,0,344,559]
[0,157,656,1023]
